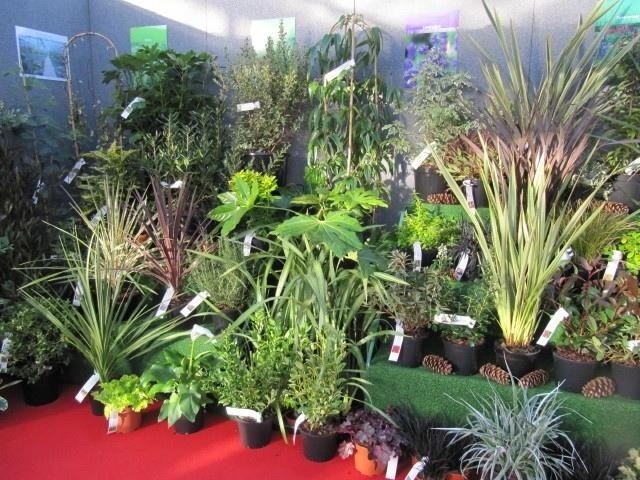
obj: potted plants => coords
[3,0,640,480]
